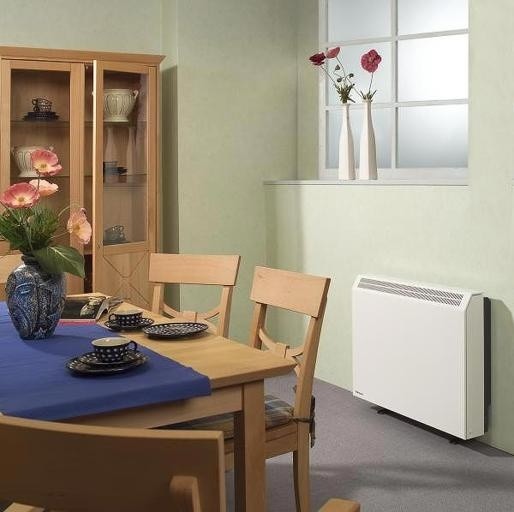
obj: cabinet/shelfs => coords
[0,45,165,312]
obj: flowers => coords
[0,146,93,280]
[309,46,381,101]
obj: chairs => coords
[0,409,229,511]
[188,263,333,511]
[143,251,245,336]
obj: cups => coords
[30,97,53,112]
[90,336,137,363]
[102,225,131,246]
[108,309,144,328]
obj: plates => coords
[22,111,61,122]
[63,349,150,375]
[103,160,127,175]
[103,318,154,332]
[141,322,209,339]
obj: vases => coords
[4,253,70,342]
[337,100,376,181]
